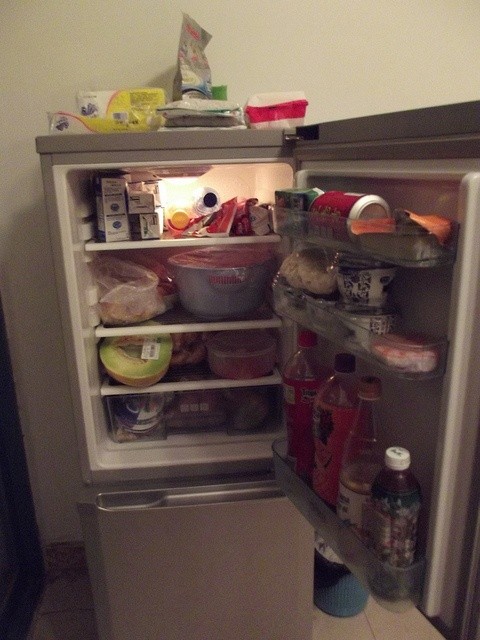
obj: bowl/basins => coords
[167,247,276,319]
[336,305,404,350]
[329,247,397,314]
[302,289,342,335]
[204,329,276,381]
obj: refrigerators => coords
[34,99,480,640]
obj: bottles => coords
[336,375,388,563]
[190,185,218,218]
[165,204,189,230]
[310,352,368,504]
[280,329,331,482]
[369,444,425,599]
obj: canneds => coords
[310,190,391,248]
